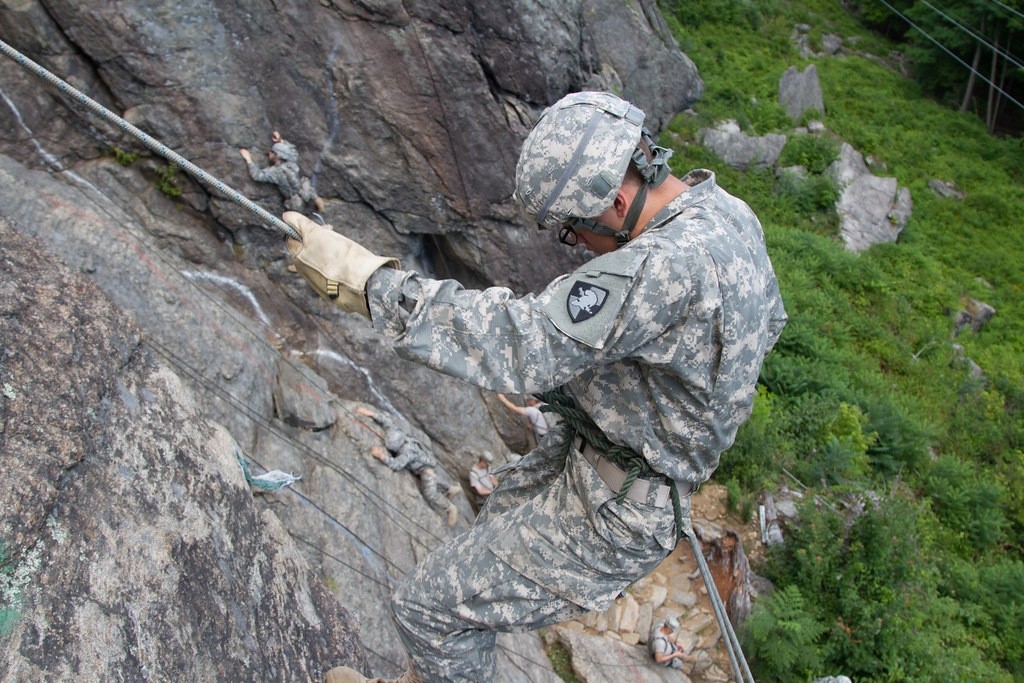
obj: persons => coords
[239,131,331,272]
[469,450,499,511]
[357,407,461,527]
[649,617,710,674]
[282,91,788,683]
[497,393,556,445]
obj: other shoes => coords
[448,486,461,501]
[679,654,697,664]
[446,503,457,527]
[314,196,324,212]
[681,666,690,675]
[287,264,297,272]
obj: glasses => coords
[559,218,579,246]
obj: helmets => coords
[837,675,852,683]
[272,143,291,160]
[385,431,405,452]
[509,454,521,463]
[665,617,679,631]
[516,92,646,232]
[526,394,539,403]
[480,450,493,463]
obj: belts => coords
[571,428,690,508]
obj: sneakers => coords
[327,658,421,683]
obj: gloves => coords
[283,211,401,322]
